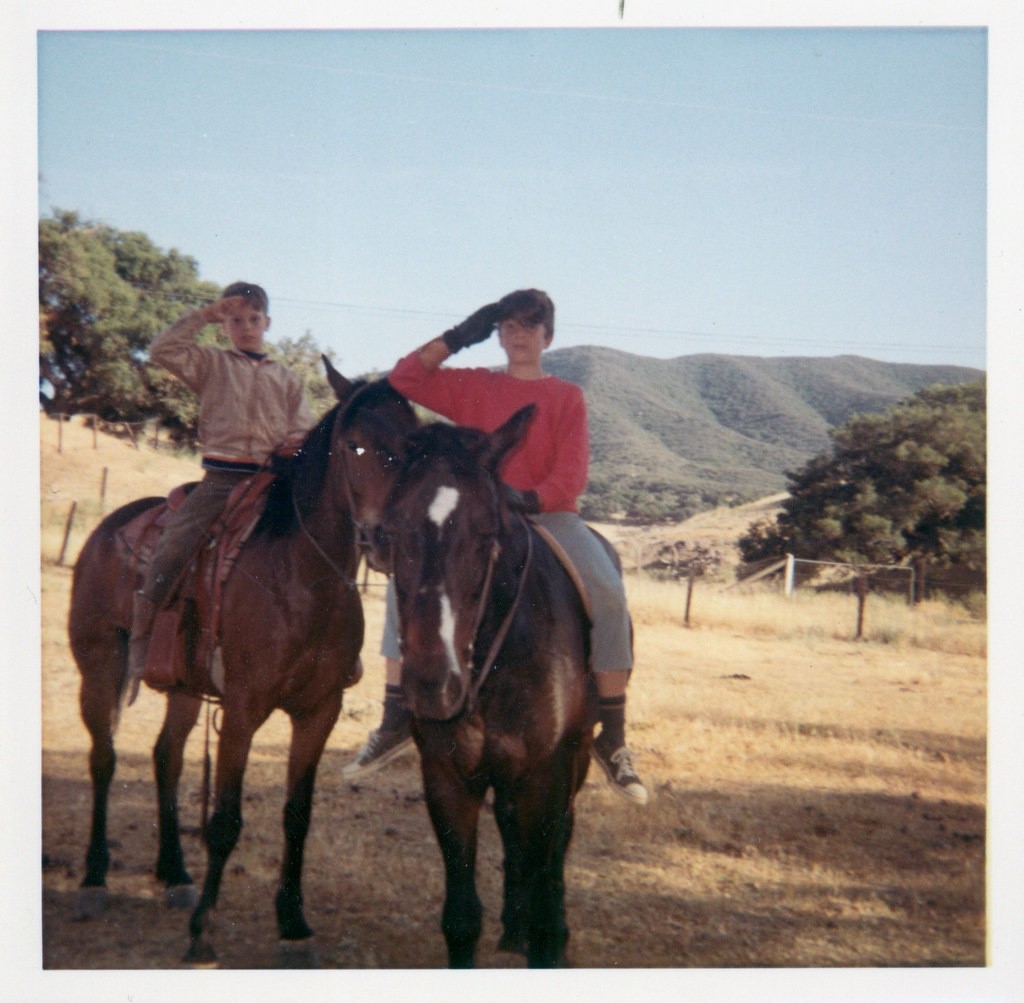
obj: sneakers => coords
[590,732,649,805]
[342,722,416,780]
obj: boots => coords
[125,591,156,674]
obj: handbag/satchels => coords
[143,609,189,693]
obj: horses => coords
[65,353,625,968]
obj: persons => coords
[126,281,316,705]
[342,288,650,805]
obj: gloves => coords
[500,483,539,514]
[442,304,512,354]
